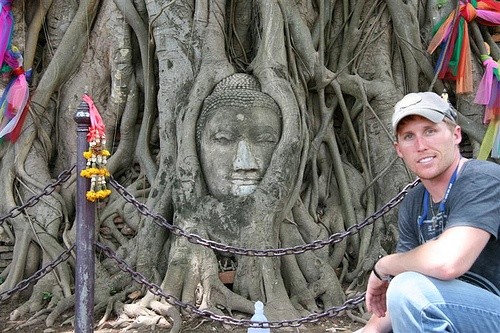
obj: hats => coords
[392,92,457,137]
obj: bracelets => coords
[372,260,394,282]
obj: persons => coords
[196,73,282,202]
[355,92,500,333]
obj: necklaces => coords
[429,194,441,231]
